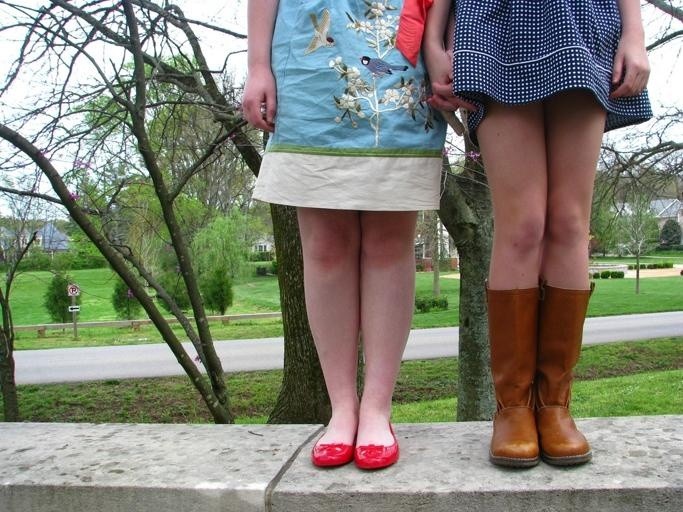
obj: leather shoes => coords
[311,419,354,466]
[355,421,399,470]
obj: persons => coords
[241,0,463,469]
[423,0,653,469]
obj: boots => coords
[540,280,595,466]
[484,276,543,468]
